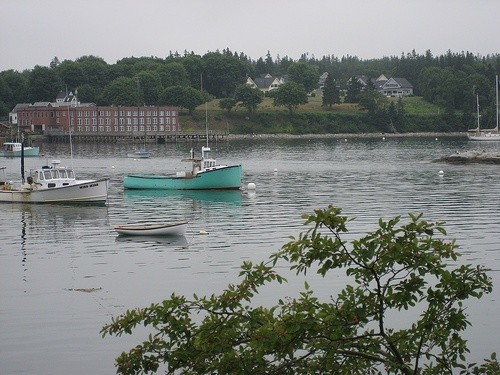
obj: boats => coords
[0,141,111,206]
[122,100,242,190]
[113,221,189,237]
[466,75,500,141]
[0,143,40,157]
